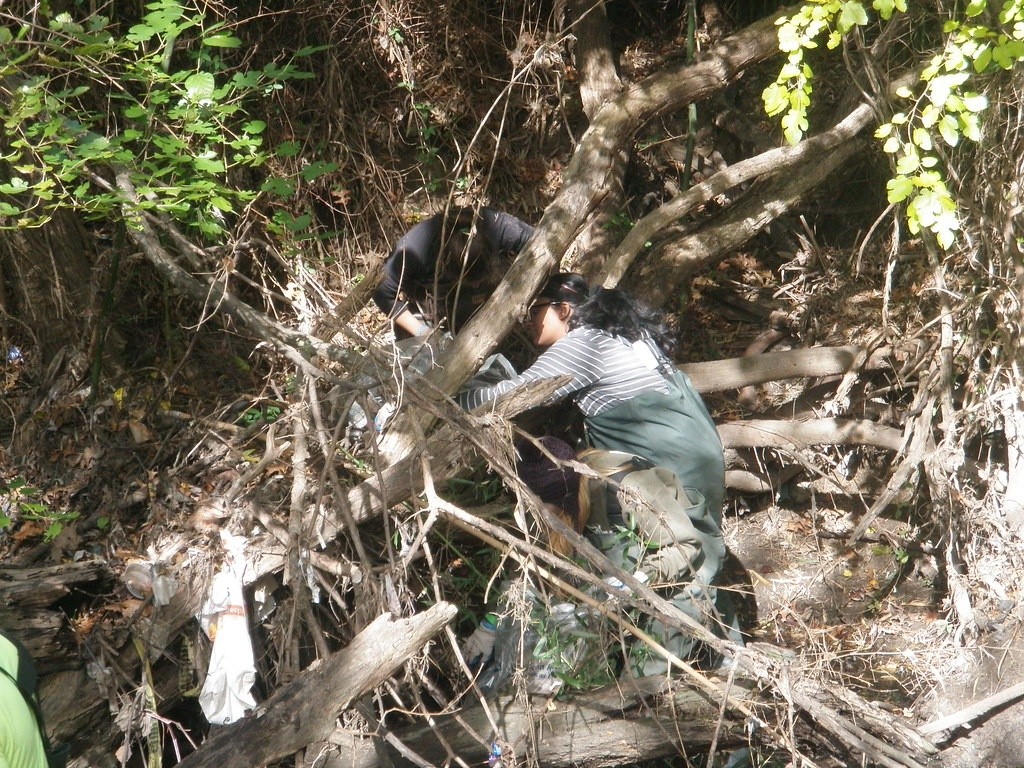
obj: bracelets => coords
[415,324,429,336]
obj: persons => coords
[372,205,536,341]
[0,630,55,768]
[516,436,762,768]
[452,272,726,528]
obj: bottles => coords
[459,611,496,668]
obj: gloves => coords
[371,400,400,436]
[450,612,499,673]
[415,326,453,351]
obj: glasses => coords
[528,300,575,314]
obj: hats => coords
[514,437,582,505]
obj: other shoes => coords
[715,743,762,767]
